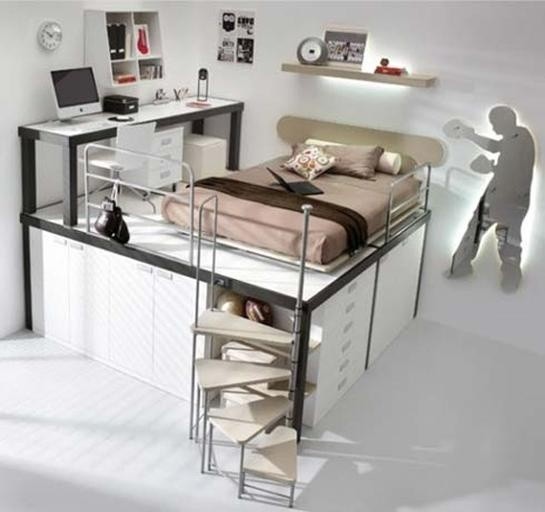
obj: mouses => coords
[116,115,129,120]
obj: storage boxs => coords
[102,94,139,115]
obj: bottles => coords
[197,68,207,103]
[155,65,161,79]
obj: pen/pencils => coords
[174,87,188,102]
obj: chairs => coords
[77,121,160,217]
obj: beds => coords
[162,114,450,273]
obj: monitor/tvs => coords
[49,65,103,124]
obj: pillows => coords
[279,142,337,185]
[281,142,387,186]
[302,138,403,176]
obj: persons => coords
[440,105,536,295]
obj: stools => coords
[184,131,226,183]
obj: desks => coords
[14,90,246,231]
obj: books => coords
[114,63,163,84]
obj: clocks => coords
[36,19,64,52]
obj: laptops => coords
[267,167,324,195]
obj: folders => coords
[107,23,126,60]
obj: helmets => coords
[95,196,130,245]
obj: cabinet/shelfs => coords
[109,123,185,203]
[362,219,429,372]
[82,5,168,107]
[262,255,379,432]
